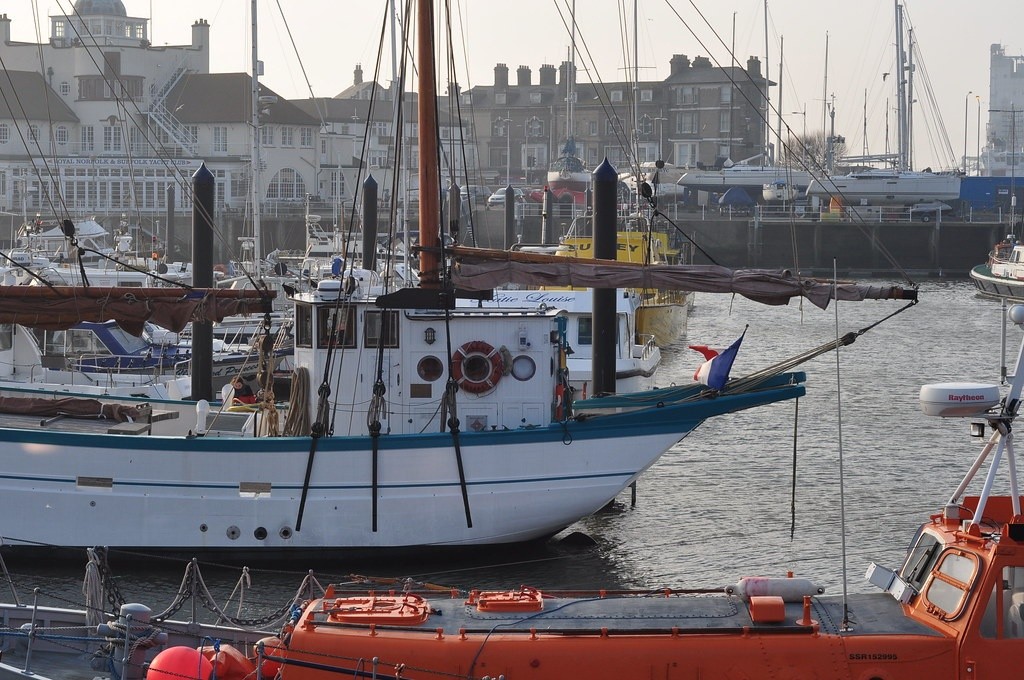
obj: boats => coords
[0,4,1024,680]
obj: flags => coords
[694,336,741,391]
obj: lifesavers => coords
[451,340,502,394]
[215,264,227,275]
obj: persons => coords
[555,384,563,423]
[222,377,256,404]
